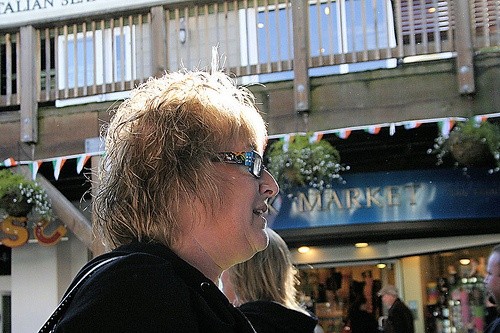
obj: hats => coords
[376,285,398,296]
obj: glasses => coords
[201,150,265,177]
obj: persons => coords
[37,68,280,332]
[220,227,319,333]
[483,244,500,333]
[377,284,414,333]
[347,297,379,333]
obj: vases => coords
[3,196,33,217]
[449,142,485,168]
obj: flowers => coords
[427,116,500,179]
[0,168,58,235]
[264,130,351,200]
